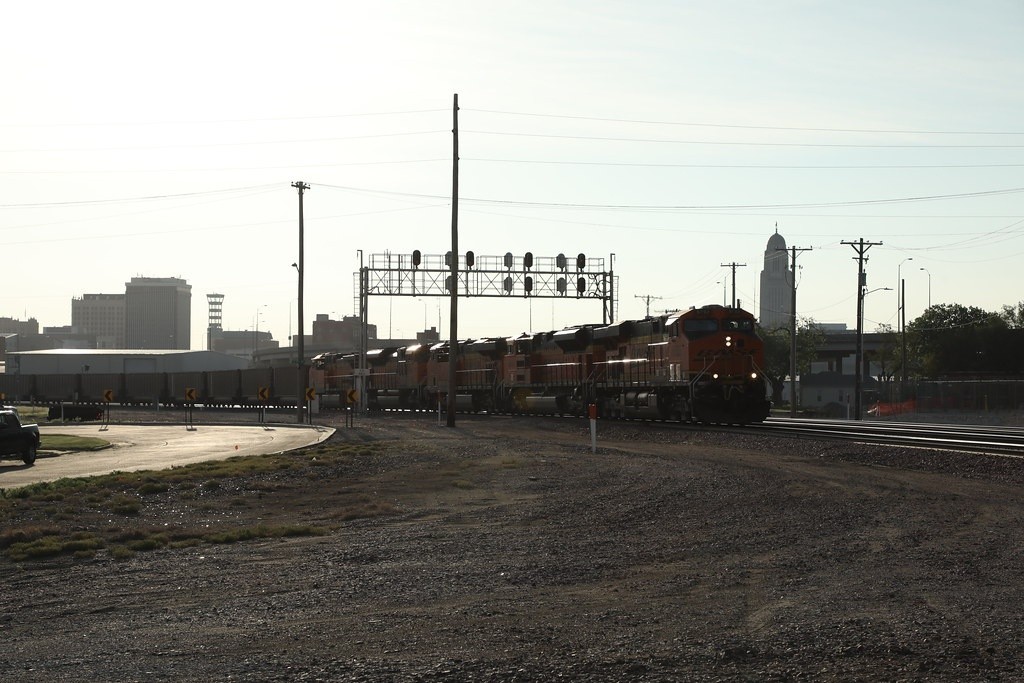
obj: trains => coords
[0,303,772,425]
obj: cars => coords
[0,411,41,465]
